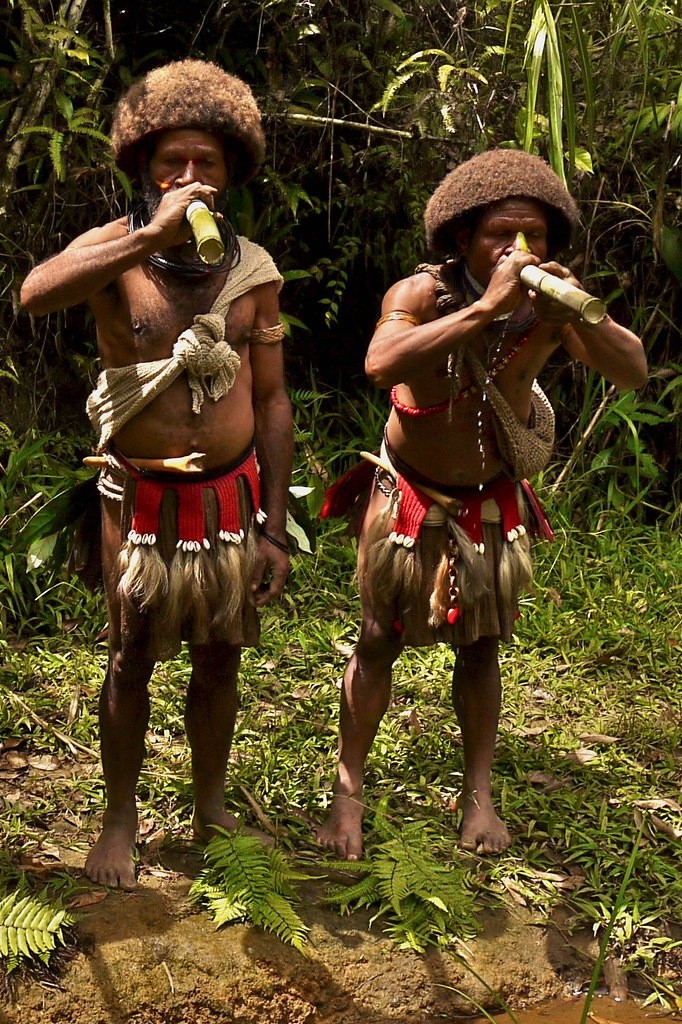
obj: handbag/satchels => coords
[462,344,555,482]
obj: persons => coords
[21,58,297,890]
[316,148,648,861]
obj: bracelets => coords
[261,526,297,557]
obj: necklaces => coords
[127,199,241,278]
[391,258,541,416]
